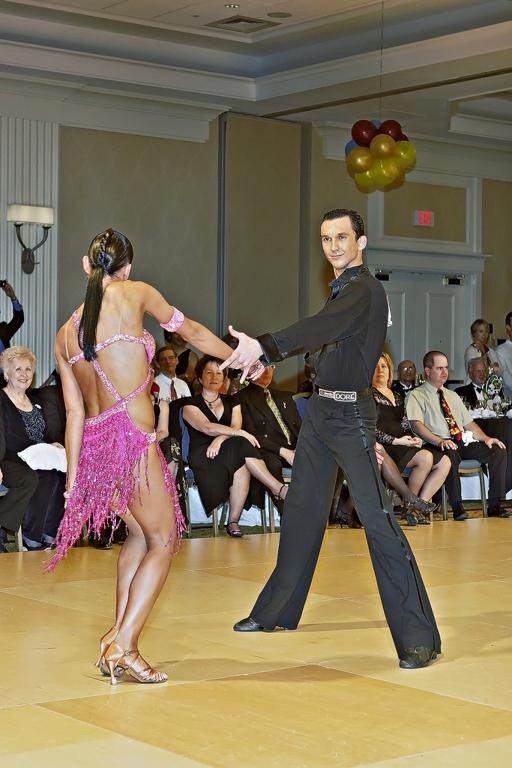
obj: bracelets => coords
[185,369,191,380]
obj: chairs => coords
[0,433,512,557]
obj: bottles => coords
[416,374,423,386]
[154,392,185,403]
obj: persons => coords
[156,328,201,395]
[0,346,129,551]
[150,334,303,537]
[463,319,501,384]
[0,280,25,352]
[336,350,512,529]
[218,210,443,668]
[489,312,512,398]
[44,226,264,687]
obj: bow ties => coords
[405,388,413,392]
[476,387,485,393]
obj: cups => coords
[459,396,509,419]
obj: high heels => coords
[94,625,169,686]
[334,508,362,528]
[400,496,440,526]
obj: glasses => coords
[399,367,415,371]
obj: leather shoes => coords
[226,521,243,537]
[487,504,512,518]
[89,527,128,549]
[24,535,56,551]
[453,506,468,521]
[234,616,263,632]
[399,646,441,668]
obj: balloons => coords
[344,118,416,194]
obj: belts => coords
[313,385,370,403]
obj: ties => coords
[437,388,462,444]
[171,380,177,401]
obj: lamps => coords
[5,202,57,274]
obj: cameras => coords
[0,280,6,287]
